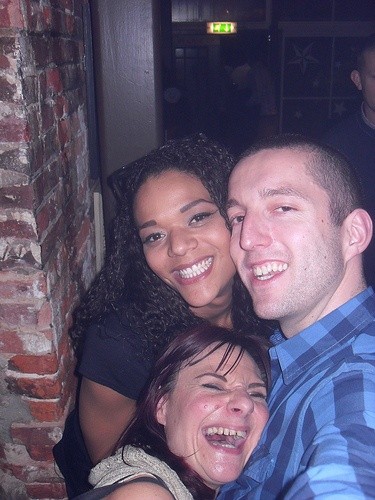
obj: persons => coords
[52,133,280,500]
[75,326,270,500]
[318,40,375,292]
[211,131,374,500]
